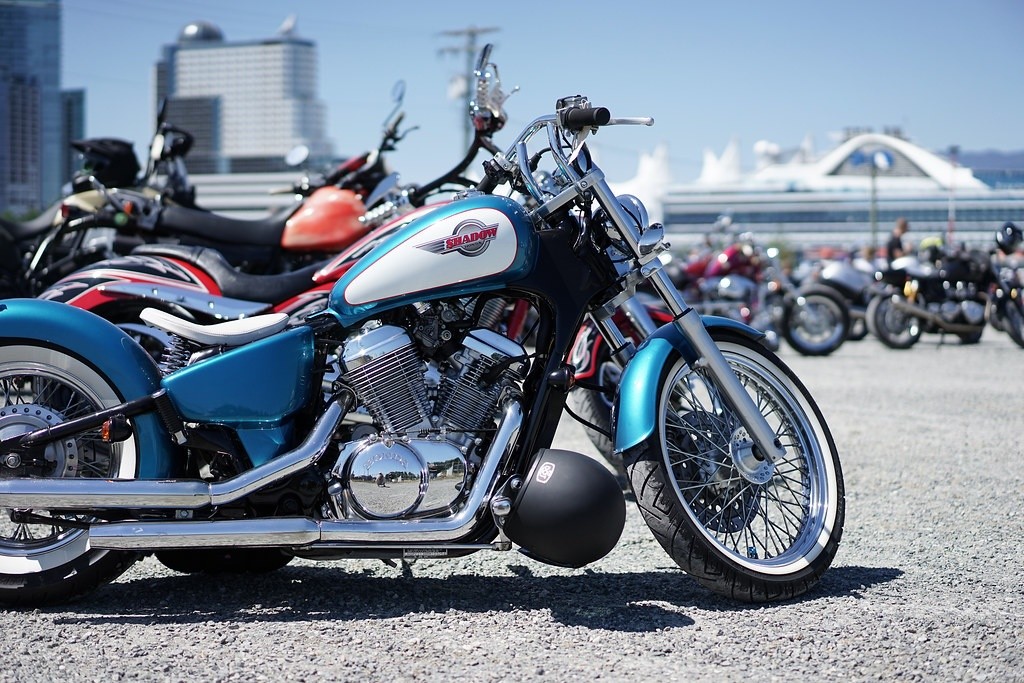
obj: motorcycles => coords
[1,39,846,611]
[2,78,422,298]
[616,206,1023,357]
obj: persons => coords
[886,218,910,268]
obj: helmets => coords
[500,448,626,569]
[69,137,140,193]
[995,222,1022,255]
[920,237,944,265]
